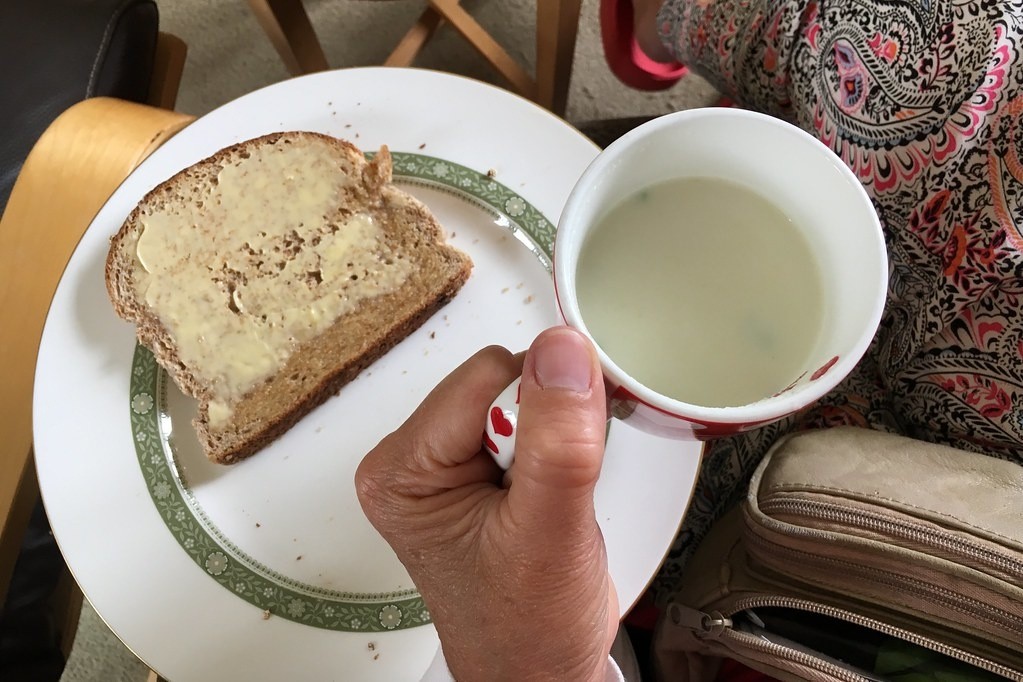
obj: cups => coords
[477,107,890,475]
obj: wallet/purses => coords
[652,424,1023,682]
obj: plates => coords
[33,63,703,681]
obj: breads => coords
[103,130,475,466]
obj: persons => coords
[355,0,1023,682]
